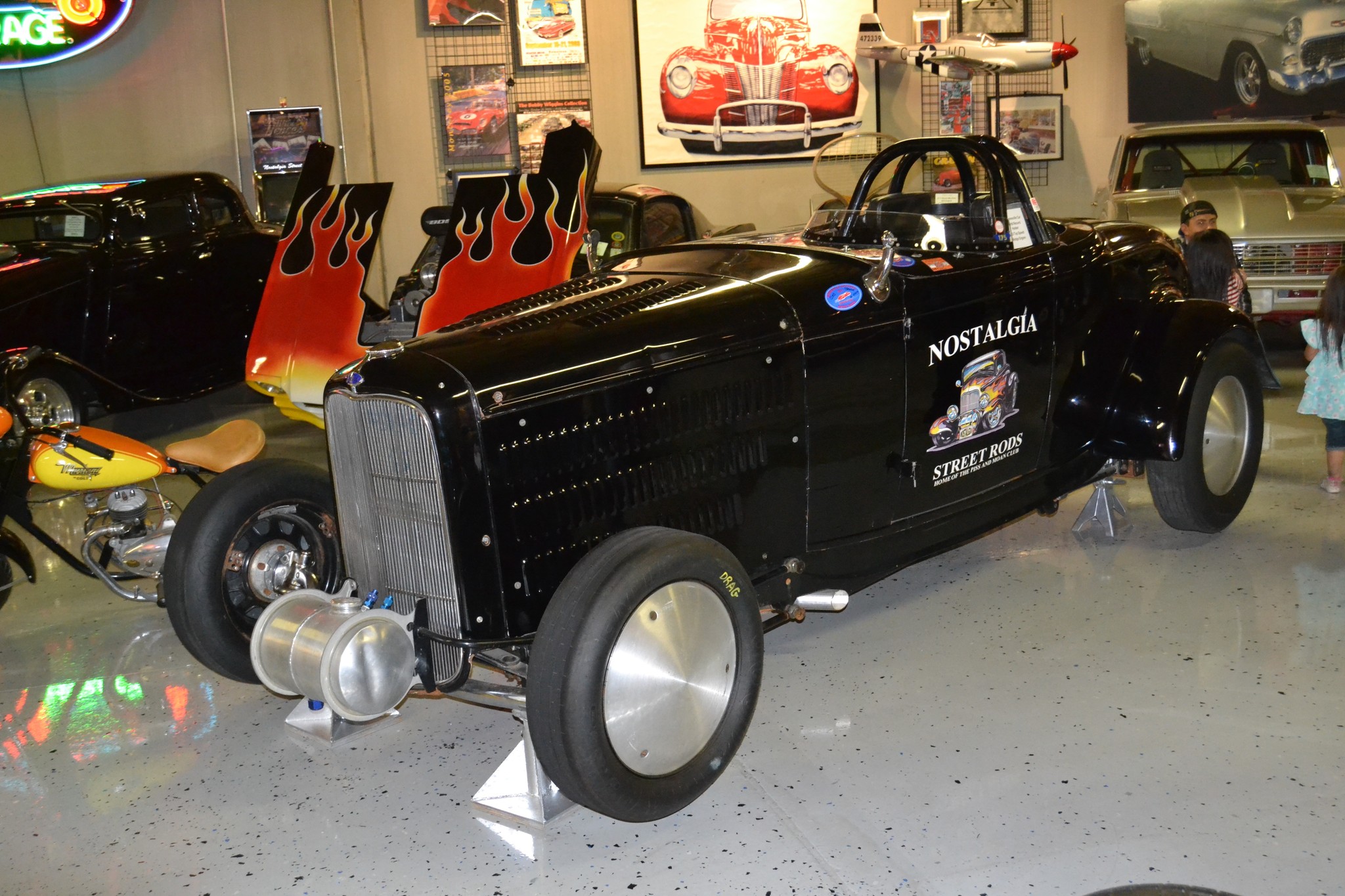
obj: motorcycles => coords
[0,346,266,613]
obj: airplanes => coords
[854,13,1079,90]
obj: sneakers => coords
[1320,476,1343,493]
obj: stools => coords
[163,418,267,473]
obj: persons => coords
[1299,265,1345,493]
[1168,199,1253,318]
[1180,228,1249,310]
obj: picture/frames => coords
[956,0,1030,38]
[911,8,949,45]
[633,0,883,171]
[939,80,972,136]
[244,105,324,225]
[986,93,1064,162]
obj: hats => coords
[1178,201,1218,236]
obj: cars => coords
[167,130,1278,824]
[1124,1,1345,110]
[655,0,864,153]
[1,170,285,440]
[1098,120,1345,357]
